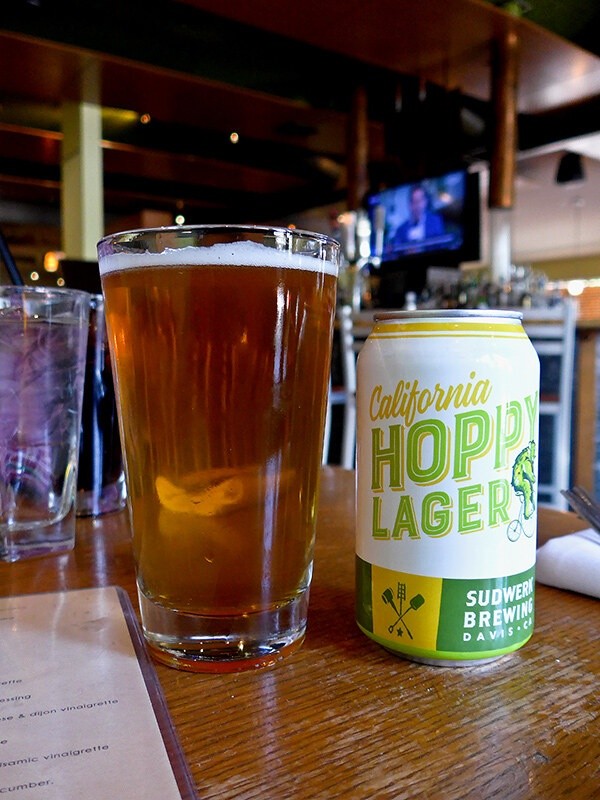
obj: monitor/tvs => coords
[363,160,490,281]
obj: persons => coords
[395,186,445,246]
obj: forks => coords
[560,486,600,537]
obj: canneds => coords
[354,308,540,668]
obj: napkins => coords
[537,528,600,597]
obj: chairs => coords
[341,296,579,511]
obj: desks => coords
[0,465,600,800]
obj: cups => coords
[0,285,91,563]
[96,223,341,675]
[34,292,128,518]
